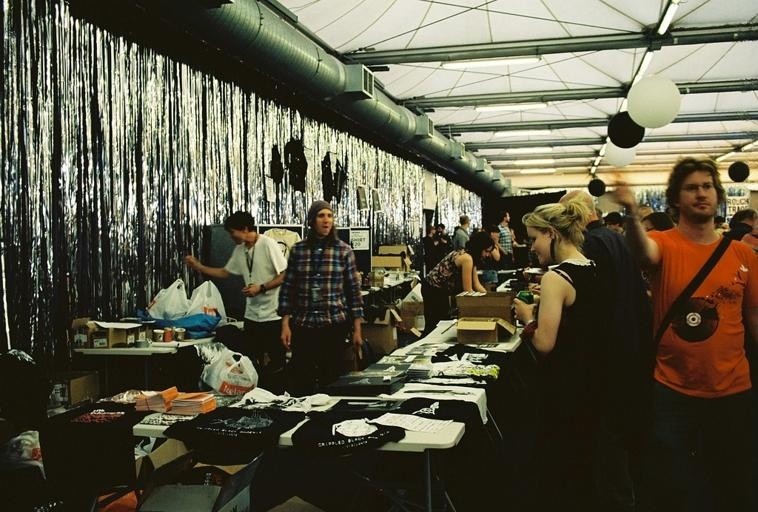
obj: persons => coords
[275,202,365,379]
[514,192,610,512]
[612,156,758,512]
[558,189,653,452]
[420,231,495,337]
[594,201,758,254]
[423,210,527,270]
[186,211,289,390]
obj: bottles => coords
[358,272,370,287]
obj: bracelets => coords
[261,283,266,295]
[520,317,538,341]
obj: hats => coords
[308,200,334,225]
[460,215,471,222]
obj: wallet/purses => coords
[513,335,539,369]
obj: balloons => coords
[586,178,606,196]
[728,161,749,183]
[608,111,645,148]
[628,75,682,129]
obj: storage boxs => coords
[372,255,411,276]
[378,243,414,258]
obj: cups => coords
[152,327,185,341]
[135,340,150,348]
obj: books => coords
[134,385,216,414]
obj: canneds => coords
[515,291,534,328]
